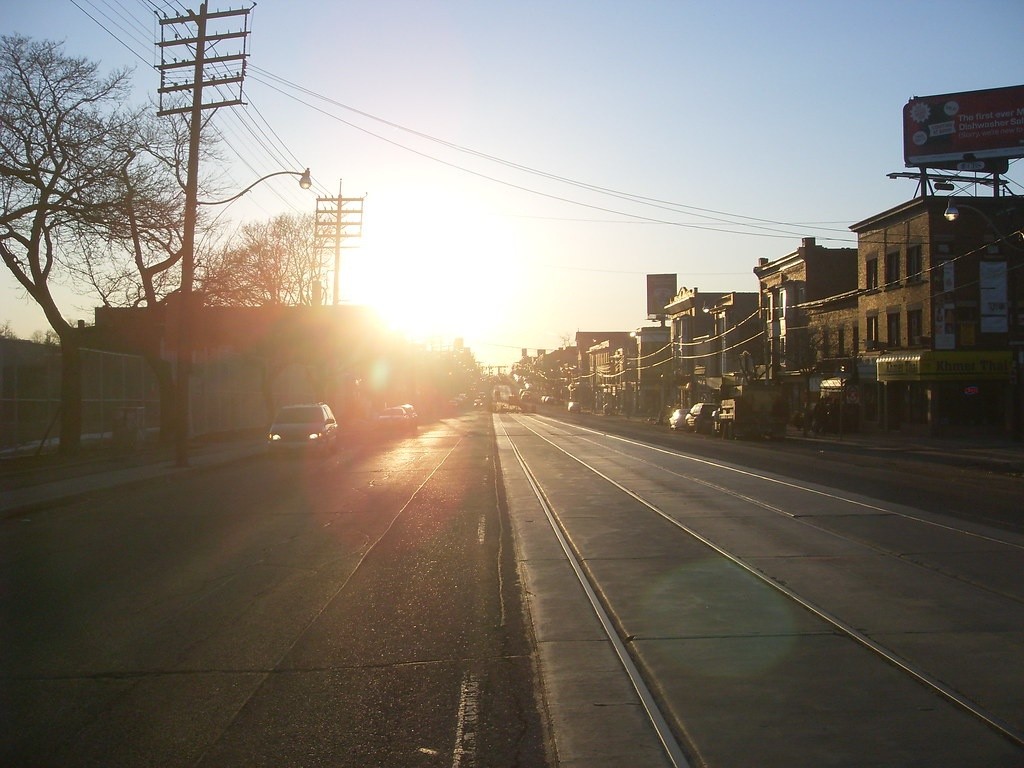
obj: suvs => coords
[684,403,720,433]
[668,408,690,432]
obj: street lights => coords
[944,197,1024,253]
[177,167,312,469]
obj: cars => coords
[541,396,554,405]
[450,392,486,411]
[568,402,581,412]
[378,404,418,429]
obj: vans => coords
[265,402,340,459]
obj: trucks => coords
[710,393,789,441]
